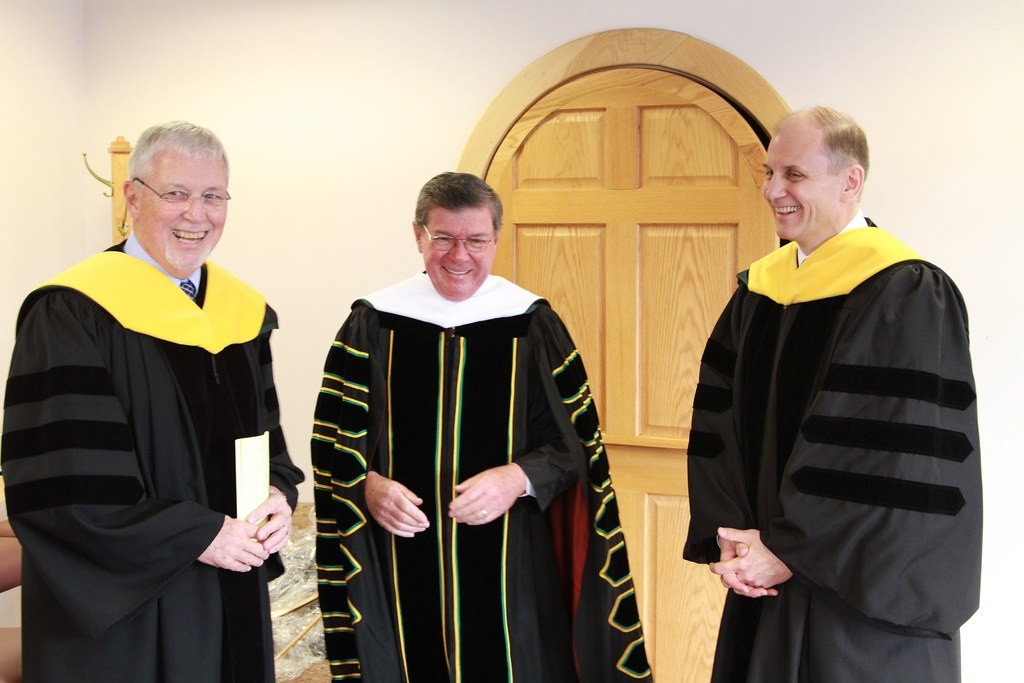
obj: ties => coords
[179,279,196,301]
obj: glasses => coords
[136,178,232,212]
[421,223,493,252]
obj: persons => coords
[0,123,304,683]
[682,107,984,683]
[310,172,653,683]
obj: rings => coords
[282,525,289,535]
[480,508,489,517]
